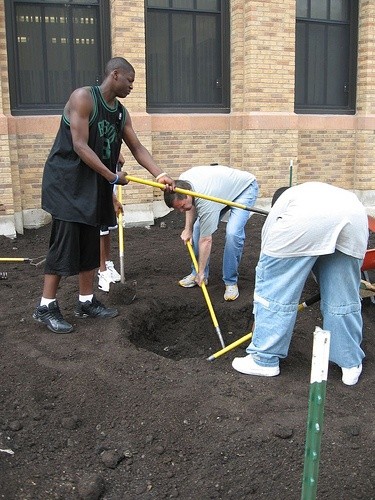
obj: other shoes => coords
[105,261,125,282]
[97,269,116,292]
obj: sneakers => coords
[232,354,280,377]
[223,284,239,301]
[178,273,208,287]
[74,295,119,320]
[33,300,73,333]
[341,363,362,386]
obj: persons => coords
[98,152,125,292]
[164,166,259,301]
[33,57,176,333]
[232,182,369,386]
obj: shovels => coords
[108,160,139,304]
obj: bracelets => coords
[109,174,118,184]
[156,173,165,179]
